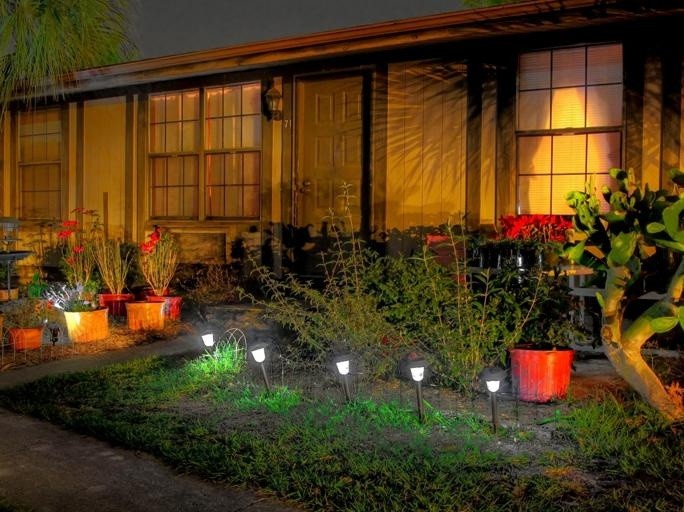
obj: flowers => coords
[500,212,597,349]
[137,225,181,296]
[50,208,103,310]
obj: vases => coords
[510,344,576,405]
[62,295,183,345]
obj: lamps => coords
[264,86,283,122]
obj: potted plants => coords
[94,235,137,318]
[1,297,48,351]
